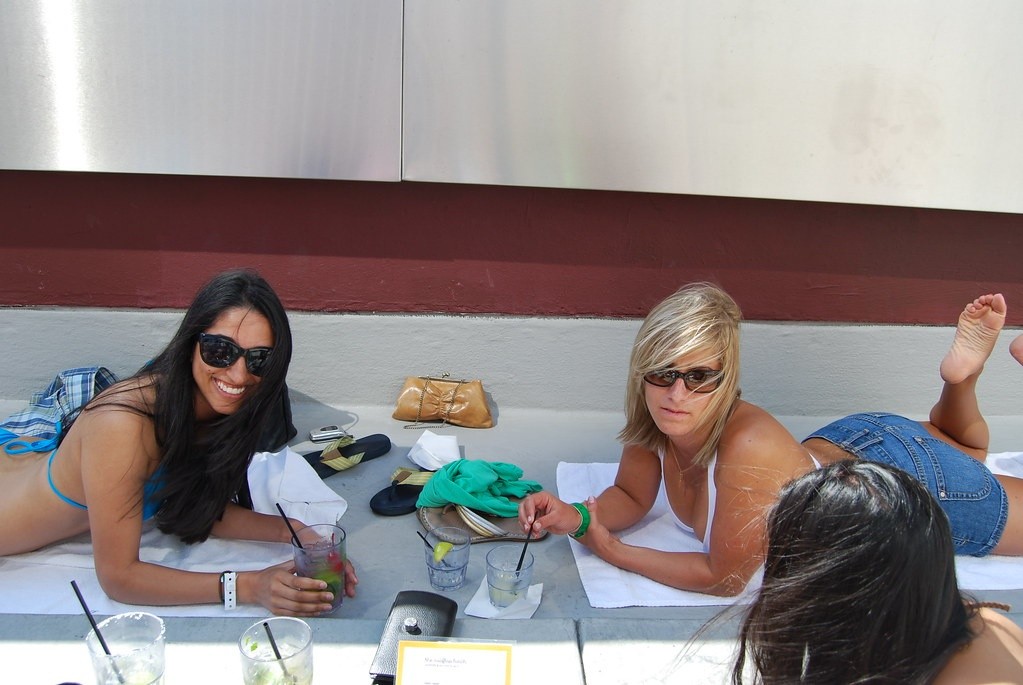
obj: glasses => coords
[198,333,272,378]
[643,364,724,394]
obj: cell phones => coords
[308,425,353,442]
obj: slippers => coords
[418,495,550,543]
[304,435,391,480]
[370,465,447,516]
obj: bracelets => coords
[219,571,238,611]
[567,502,591,540]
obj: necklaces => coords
[668,439,707,488]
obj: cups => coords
[422,526,471,592]
[291,523,346,616]
[485,545,535,611]
[85,611,166,685]
[236,615,314,685]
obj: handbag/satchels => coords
[1,366,122,439]
[391,375,493,429]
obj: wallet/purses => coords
[370,590,458,683]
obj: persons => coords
[731,462,1023,685]
[518,282,1023,597]
[0,266,357,615]
